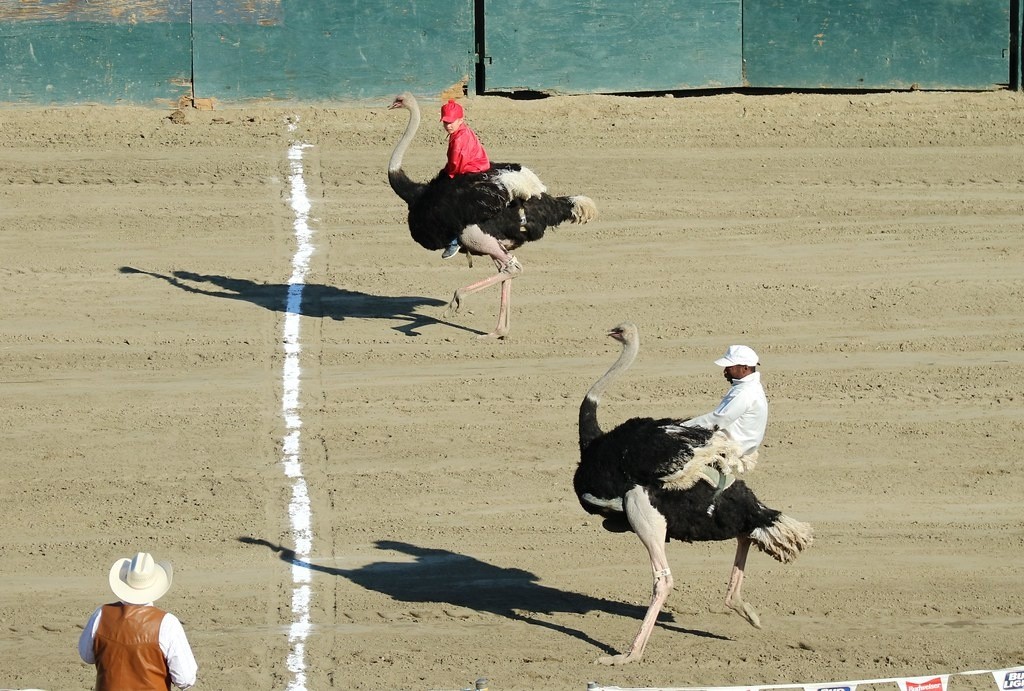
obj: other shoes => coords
[583,494,624,511]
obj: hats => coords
[715,345,759,366]
[109,553,173,604]
[439,100,464,122]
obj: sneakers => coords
[442,243,463,259]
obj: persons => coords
[425,99,491,259]
[77,550,199,690]
[681,344,769,490]
